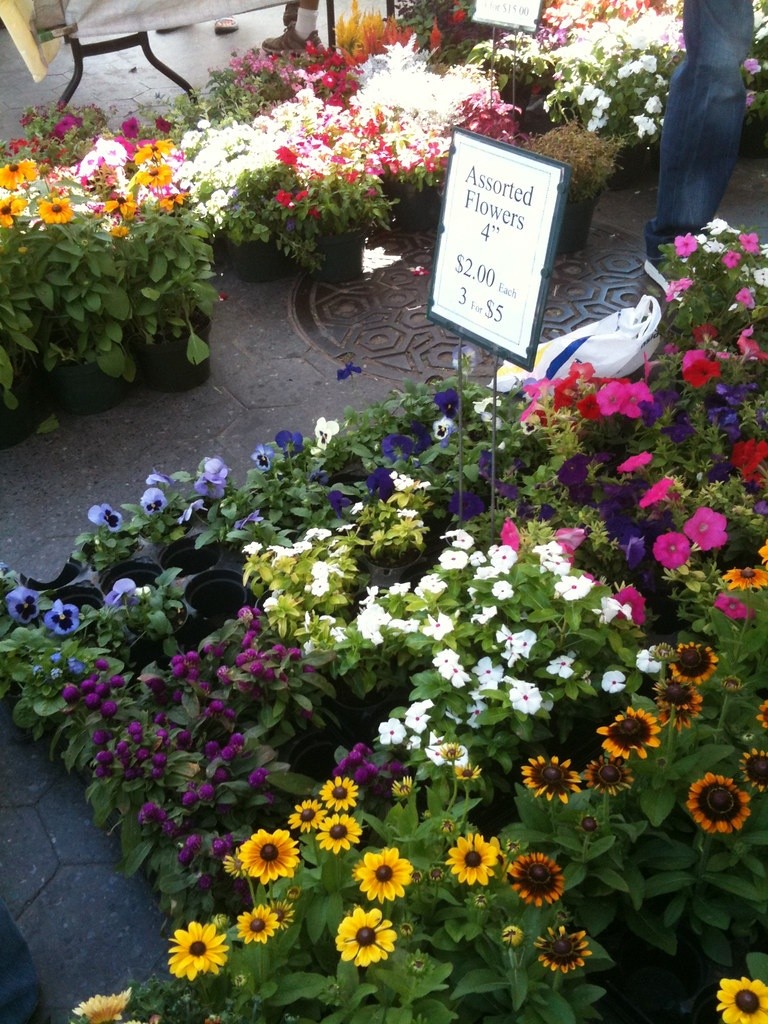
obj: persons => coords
[262,0,326,56]
[644,0,754,302]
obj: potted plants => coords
[519,123,625,253]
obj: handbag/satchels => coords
[488,294,666,396]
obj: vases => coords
[190,494,225,532]
[329,679,392,734]
[604,144,637,191]
[125,595,190,662]
[161,533,222,582]
[98,554,170,612]
[81,528,146,579]
[0,358,43,450]
[443,519,458,547]
[137,506,199,555]
[328,466,368,502]
[738,118,766,156]
[131,309,211,391]
[596,441,628,478]
[305,233,363,283]
[220,230,293,282]
[610,933,707,1024]
[498,73,538,120]
[44,330,131,415]
[380,179,443,230]
[365,542,422,586]
[183,568,252,640]
[19,554,88,592]
[40,579,107,624]
[290,740,335,784]
[688,977,723,1024]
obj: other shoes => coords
[645,255,701,303]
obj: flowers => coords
[1,0,768,1024]
